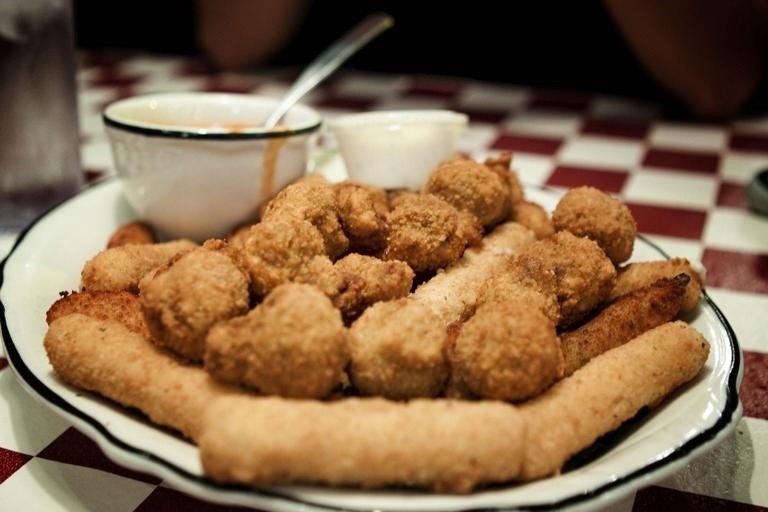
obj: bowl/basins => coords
[99,89,326,251]
[324,104,473,196]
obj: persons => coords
[195,2,767,125]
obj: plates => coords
[0,137,750,512]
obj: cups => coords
[0,0,88,235]
